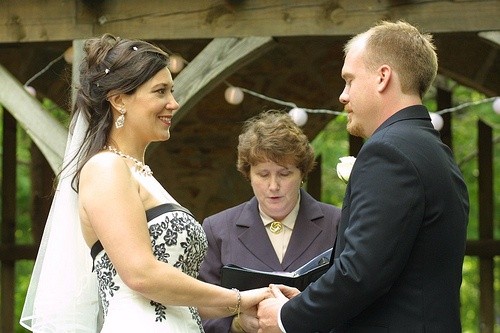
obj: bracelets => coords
[226,287,240,318]
[234,315,247,333]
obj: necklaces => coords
[102,144,152,176]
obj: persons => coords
[256,21,471,333]
[194,110,341,333]
[19,33,274,333]
